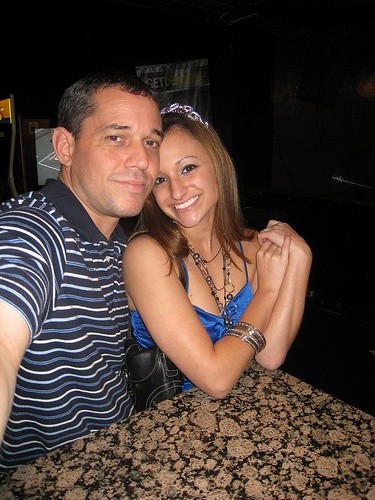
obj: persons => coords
[180,230,239,332]
[1,69,166,476]
[121,106,311,409]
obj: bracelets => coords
[223,322,267,354]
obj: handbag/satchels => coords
[124,337,184,412]
